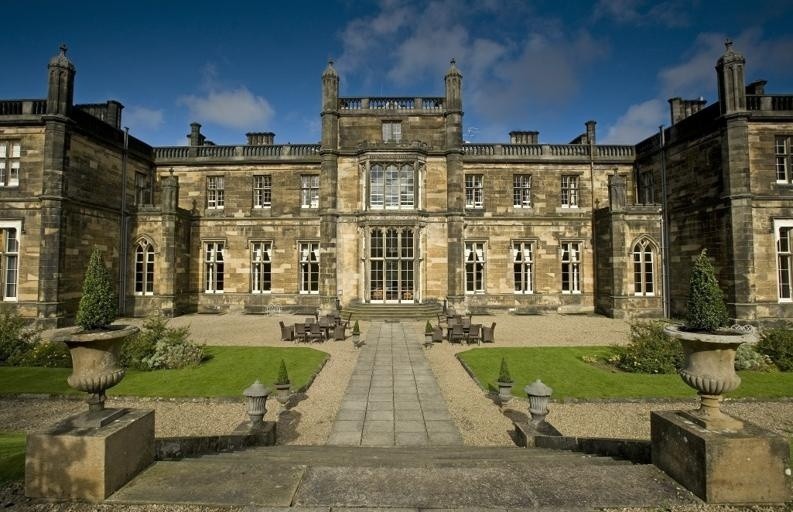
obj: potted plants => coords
[492,357,515,408]
[663,248,760,432]
[54,247,140,427]
[424,320,435,346]
[351,319,361,347]
[272,358,291,415]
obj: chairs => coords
[279,309,353,344]
[432,309,496,346]
